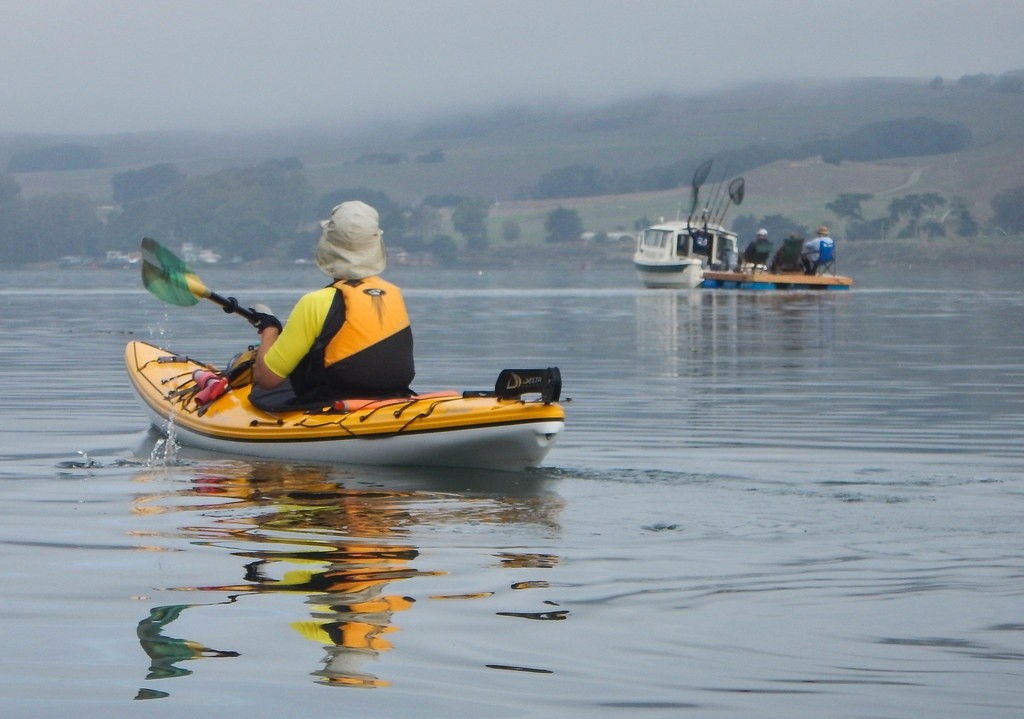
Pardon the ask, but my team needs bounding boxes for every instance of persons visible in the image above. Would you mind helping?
[800,226,834,275]
[770,233,800,272]
[244,199,415,412]
[738,228,770,272]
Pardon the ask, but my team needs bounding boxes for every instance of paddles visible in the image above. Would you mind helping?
[139,236,257,322]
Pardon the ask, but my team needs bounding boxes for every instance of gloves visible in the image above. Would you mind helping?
[246,303,283,335]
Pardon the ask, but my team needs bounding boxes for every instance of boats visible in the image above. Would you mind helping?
[632,220,740,288]
[696,256,855,295]
[121,337,568,471]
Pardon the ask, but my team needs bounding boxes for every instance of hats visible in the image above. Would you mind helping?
[315,201,386,280]
[757,229,768,236]
[816,227,828,234]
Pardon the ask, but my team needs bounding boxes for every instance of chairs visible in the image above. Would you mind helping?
[733,251,771,272]
[769,236,805,275]
[801,241,836,277]
